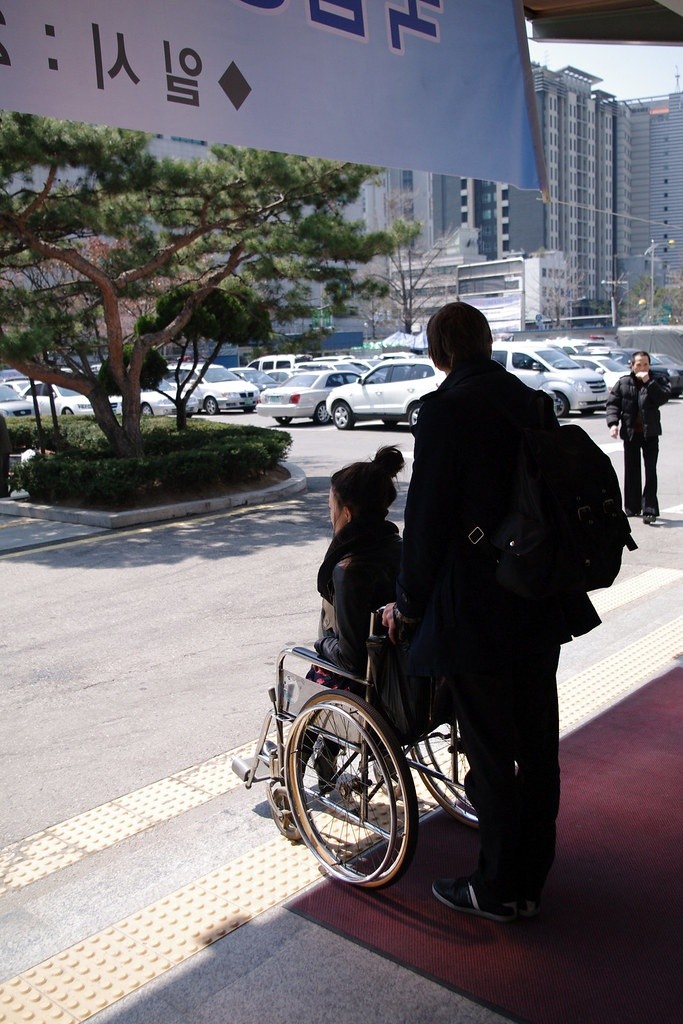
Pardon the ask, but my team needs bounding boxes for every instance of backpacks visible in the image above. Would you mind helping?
[498,392,636,598]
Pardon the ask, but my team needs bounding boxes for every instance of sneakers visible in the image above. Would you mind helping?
[431,875,515,921]
[516,899,540,916]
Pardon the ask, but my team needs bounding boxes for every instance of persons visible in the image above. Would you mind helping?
[606,352,671,524]
[258,447,404,791]
[379,302,562,920]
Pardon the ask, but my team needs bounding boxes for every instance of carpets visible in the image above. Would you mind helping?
[282,667,683,1024]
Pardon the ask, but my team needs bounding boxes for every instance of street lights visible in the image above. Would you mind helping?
[639,239,675,308]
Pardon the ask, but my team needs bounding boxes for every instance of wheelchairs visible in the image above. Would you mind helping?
[231,604,523,891]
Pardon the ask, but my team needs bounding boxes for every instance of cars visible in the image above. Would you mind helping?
[256,369,361,425]
[0,337,682,420]
[325,356,448,429]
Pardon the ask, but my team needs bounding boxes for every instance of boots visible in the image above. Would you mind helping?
[261,740,315,785]
[311,736,339,795]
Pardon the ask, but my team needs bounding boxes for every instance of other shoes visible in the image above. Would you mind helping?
[642,513,656,524]
